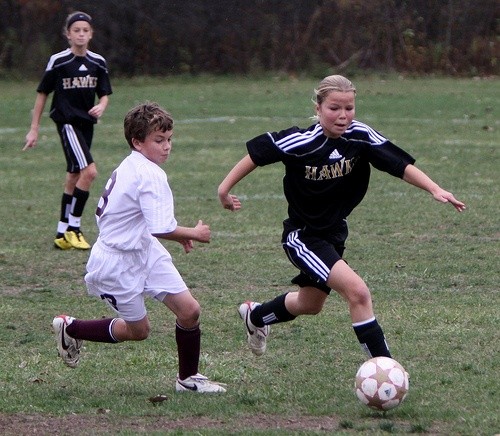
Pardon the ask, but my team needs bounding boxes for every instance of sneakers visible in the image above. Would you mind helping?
[54,234,76,250]
[63,229,93,249]
[175,370,227,393]
[52,314,81,368]
[239,300,270,356]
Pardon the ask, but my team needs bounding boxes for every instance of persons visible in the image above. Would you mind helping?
[52,102,229,394]
[218,74,465,383]
[21,10,113,252]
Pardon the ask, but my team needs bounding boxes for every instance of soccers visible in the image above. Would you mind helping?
[354,355,409,412]
